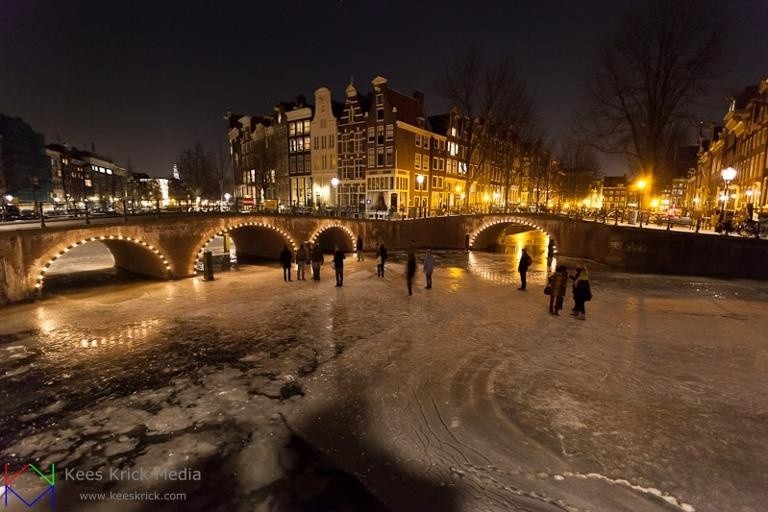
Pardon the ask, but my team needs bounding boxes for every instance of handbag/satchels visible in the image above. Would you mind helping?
[544,287,552,295]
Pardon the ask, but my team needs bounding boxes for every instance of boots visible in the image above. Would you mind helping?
[571,311,585,320]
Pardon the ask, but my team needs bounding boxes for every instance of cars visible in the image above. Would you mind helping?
[0,203,49,222]
[607,209,692,225]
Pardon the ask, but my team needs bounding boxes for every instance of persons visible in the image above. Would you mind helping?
[548,238,554,257]
[406,254,415,296]
[408,240,416,256]
[548,261,593,320]
[280,235,387,286]
[517,249,528,291]
[423,250,434,288]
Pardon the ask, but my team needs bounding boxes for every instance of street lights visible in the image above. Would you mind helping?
[331,177,339,217]
[416,175,425,218]
[714,164,737,233]
